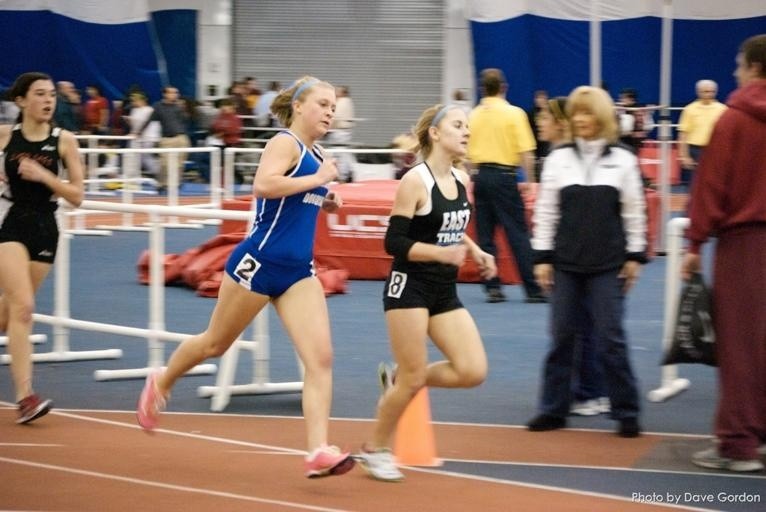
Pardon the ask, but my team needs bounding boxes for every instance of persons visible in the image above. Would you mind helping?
[1,72,84,425]
[680,34,766,474]
[2,78,353,194]
[467,69,546,304]
[679,79,730,184]
[527,86,649,438]
[538,95,608,417]
[139,74,359,480]
[356,104,498,484]
[619,89,654,143]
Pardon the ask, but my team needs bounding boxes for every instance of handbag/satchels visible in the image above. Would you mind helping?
[661,272,718,368]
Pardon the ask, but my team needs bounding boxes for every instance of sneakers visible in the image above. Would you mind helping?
[525,292,551,302]
[529,397,766,472]
[486,288,506,303]
[15,395,55,424]
[137,368,171,429]
[303,361,406,482]
